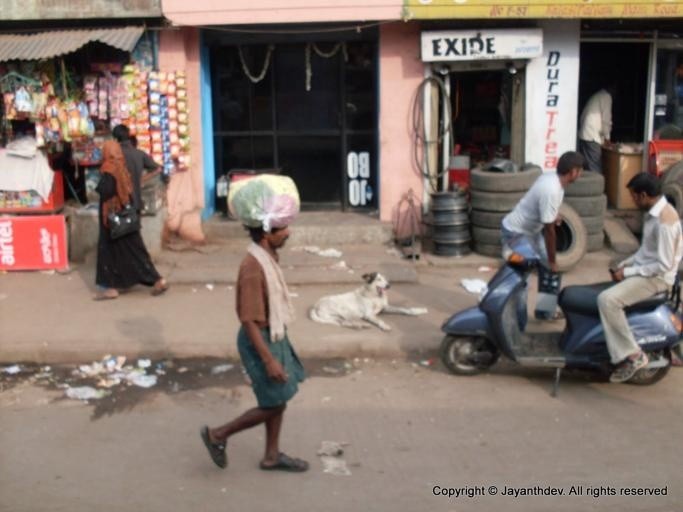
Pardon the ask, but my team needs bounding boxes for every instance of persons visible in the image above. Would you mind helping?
[93,125,168,300]
[579,81,621,173]
[48,131,79,201]
[597,172,683,383]
[502,151,585,319]
[199,224,308,472]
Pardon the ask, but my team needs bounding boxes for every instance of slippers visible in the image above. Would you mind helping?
[92,291,117,300]
[150,283,168,296]
[201,424,228,470]
[259,453,308,471]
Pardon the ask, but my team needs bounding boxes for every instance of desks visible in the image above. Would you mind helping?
[0,212,72,270]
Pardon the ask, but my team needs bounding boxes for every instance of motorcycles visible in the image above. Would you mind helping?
[435,233,681,386]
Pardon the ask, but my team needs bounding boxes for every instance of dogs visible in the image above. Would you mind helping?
[310,272,428,332]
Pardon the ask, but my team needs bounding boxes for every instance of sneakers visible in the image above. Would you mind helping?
[609,352,648,383]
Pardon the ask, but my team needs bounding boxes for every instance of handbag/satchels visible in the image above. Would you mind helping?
[107,204,140,239]
